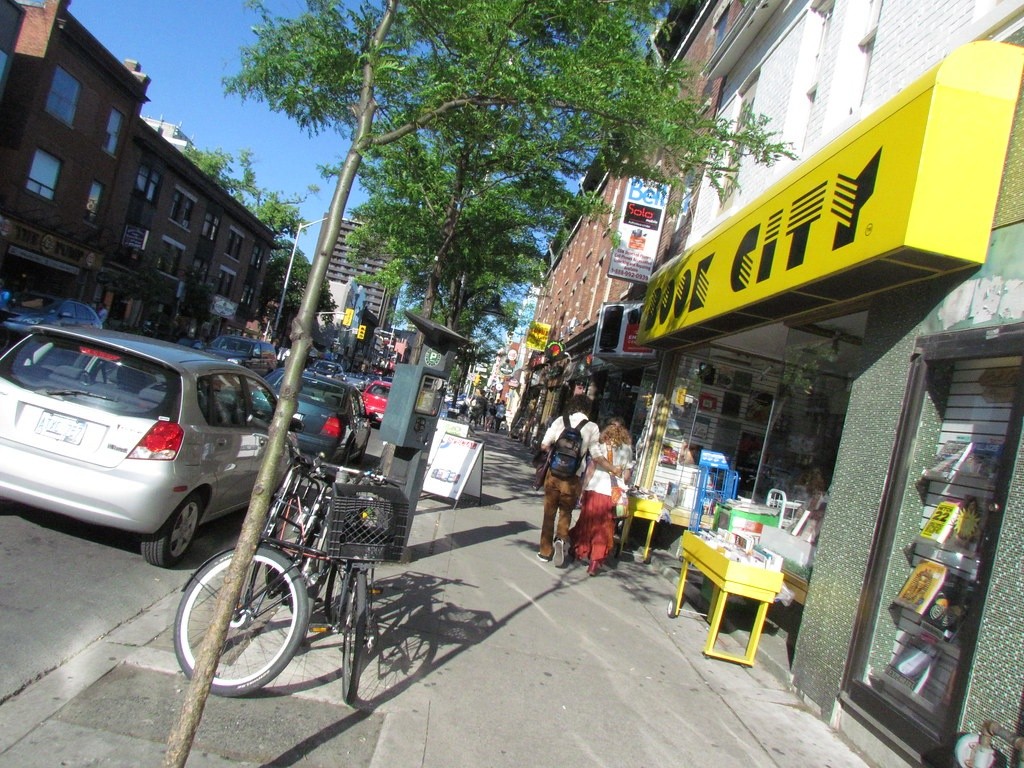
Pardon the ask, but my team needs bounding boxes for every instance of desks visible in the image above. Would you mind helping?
[614,494,663,563]
[668,530,785,668]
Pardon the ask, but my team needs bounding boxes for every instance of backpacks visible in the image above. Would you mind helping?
[549,413,590,480]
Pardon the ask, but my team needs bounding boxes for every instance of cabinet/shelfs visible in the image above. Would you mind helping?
[871,467,998,714]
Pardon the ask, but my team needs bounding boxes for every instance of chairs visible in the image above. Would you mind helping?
[298,385,341,406]
[49,365,166,410]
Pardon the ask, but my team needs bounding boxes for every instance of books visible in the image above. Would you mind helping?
[657,386,717,469]
[895,440,1005,642]
[694,528,784,573]
[791,508,824,542]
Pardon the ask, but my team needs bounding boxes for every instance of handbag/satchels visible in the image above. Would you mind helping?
[489,405,496,415]
[501,416,506,421]
[616,491,628,517]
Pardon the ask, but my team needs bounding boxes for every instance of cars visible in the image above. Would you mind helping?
[348,367,468,416]
[359,380,393,426]
[204,334,278,378]
[0,325,305,568]
[0,291,102,345]
[306,360,346,384]
[263,368,371,469]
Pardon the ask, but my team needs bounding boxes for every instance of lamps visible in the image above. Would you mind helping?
[756,365,774,382]
[826,329,841,363]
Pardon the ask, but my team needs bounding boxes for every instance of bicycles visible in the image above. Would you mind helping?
[172,431,333,698]
[312,455,408,705]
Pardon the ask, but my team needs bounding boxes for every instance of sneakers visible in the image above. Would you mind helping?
[537,552,548,562]
[553,538,564,567]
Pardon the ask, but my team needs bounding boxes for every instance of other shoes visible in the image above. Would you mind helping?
[487,429,489,431]
[484,429,486,431]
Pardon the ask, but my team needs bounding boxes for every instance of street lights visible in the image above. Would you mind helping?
[446,291,507,416]
[273,217,348,336]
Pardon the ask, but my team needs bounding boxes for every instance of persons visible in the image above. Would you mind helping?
[476,390,506,433]
[536,394,623,567]
[97,302,108,323]
[569,417,636,575]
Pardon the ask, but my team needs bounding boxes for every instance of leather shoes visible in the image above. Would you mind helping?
[587,560,598,576]
